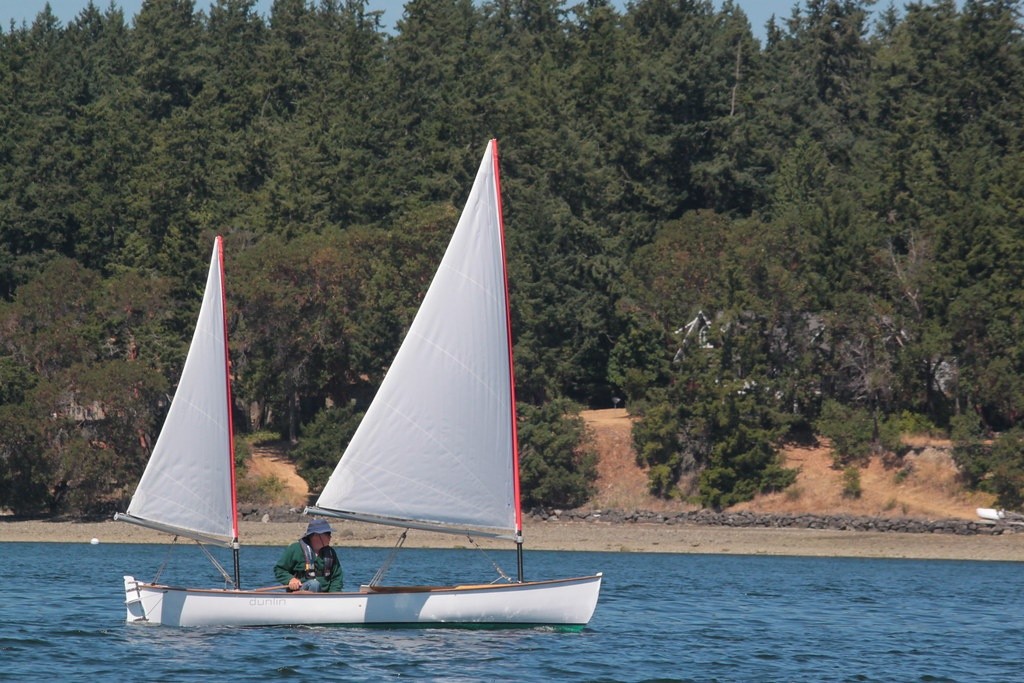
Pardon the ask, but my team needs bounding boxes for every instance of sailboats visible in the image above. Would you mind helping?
[110,139,601,638]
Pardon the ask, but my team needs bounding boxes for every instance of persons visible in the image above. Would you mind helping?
[274,517,344,594]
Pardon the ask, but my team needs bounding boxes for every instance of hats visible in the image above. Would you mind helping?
[297,519,337,540]
